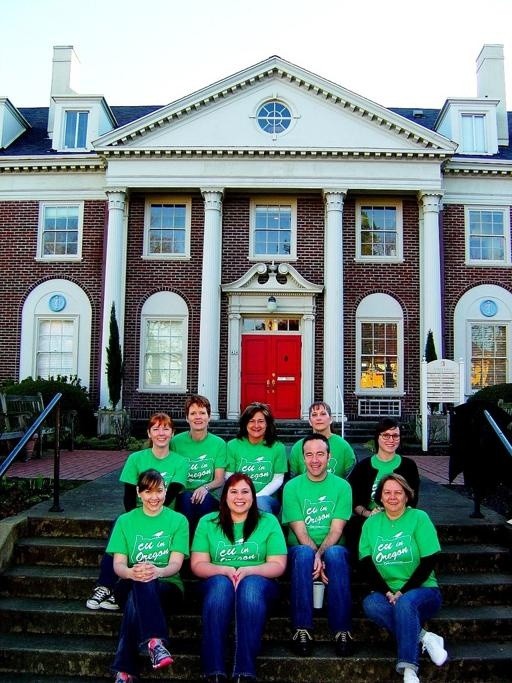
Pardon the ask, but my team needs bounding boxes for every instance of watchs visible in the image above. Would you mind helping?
[202,484,211,492]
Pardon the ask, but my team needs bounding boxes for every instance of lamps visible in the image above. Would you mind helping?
[255,259,289,285]
[266,295,278,314]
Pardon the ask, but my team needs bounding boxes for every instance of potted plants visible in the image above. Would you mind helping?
[414,328,450,445]
[94,300,131,439]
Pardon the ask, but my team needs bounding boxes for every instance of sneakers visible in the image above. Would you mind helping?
[115,672,132,682]
[293,629,312,658]
[87,586,110,610]
[148,637,174,668]
[403,668,421,682]
[421,632,449,666]
[335,630,354,657]
[100,595,121,611]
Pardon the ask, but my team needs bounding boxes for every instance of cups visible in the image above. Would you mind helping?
[138,562,150,582]
[313,581,325,609]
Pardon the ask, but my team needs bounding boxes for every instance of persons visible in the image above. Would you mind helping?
[345,419,421,550]
[357,472,449,683]
[289,401,357,478]
[279,433,356,656]
[216,400,288,516]
[86,410,189,609]
[170,392,227,542]
[103,470,190,683]
[187,473,288,682]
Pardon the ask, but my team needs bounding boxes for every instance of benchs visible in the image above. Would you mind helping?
[0,390,78,457]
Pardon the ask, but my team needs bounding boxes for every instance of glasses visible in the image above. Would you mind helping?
[378,432,398,441]
